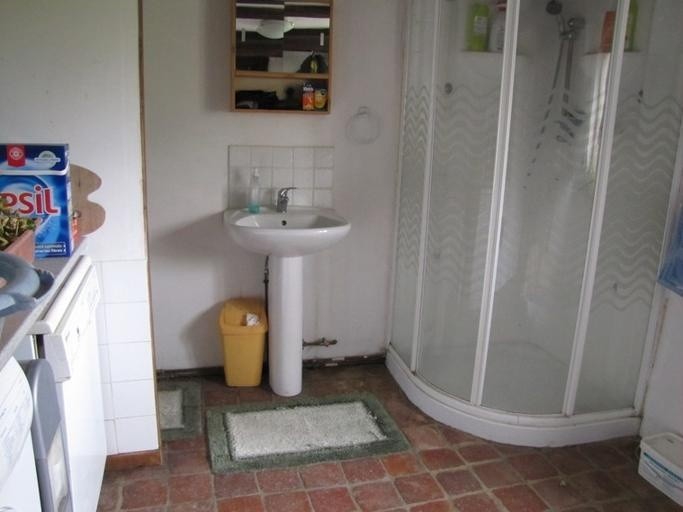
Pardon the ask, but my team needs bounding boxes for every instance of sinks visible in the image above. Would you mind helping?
[223,205,352,258]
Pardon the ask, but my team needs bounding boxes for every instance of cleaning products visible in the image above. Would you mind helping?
[488,0,507,53]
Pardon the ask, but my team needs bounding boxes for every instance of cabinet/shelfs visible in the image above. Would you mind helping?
[227,0,332,116]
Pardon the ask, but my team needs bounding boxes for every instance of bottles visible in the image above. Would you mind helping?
[248,168,260,214]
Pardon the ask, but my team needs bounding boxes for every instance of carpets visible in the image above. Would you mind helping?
[157,377,411,476]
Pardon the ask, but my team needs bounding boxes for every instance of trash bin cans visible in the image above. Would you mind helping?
[217,296,270,389]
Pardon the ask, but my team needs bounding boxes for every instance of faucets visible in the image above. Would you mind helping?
[277,187,298,213]
